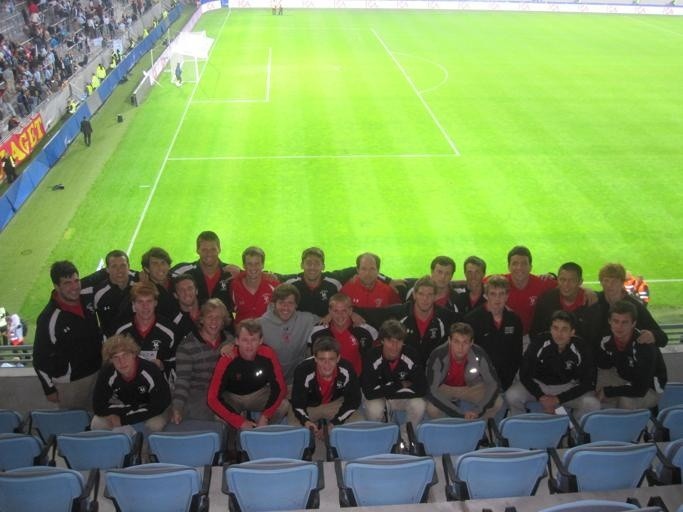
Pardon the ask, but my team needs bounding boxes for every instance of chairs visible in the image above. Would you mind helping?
[0,382,682,511]
[0,1,161,149]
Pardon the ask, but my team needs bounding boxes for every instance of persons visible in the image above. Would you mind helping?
[272,0,277,15]
[0,0,183,192]
[278,0,283,15]
